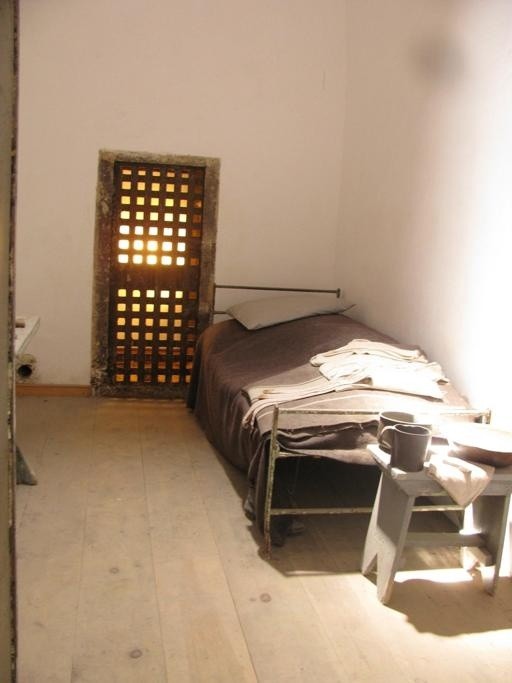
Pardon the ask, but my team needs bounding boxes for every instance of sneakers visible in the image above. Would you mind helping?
[244,486,305,534]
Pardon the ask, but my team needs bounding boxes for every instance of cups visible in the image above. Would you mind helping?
[378,423,432,472]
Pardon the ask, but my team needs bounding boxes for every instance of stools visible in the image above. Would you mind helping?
[361,442,512,603]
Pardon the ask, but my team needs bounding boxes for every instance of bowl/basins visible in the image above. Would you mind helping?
[378,411,432,451]
[446,422,512,467]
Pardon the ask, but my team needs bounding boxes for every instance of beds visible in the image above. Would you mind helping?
[185,281,493,560]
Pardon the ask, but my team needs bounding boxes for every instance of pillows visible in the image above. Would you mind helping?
[224,292,356,331]
